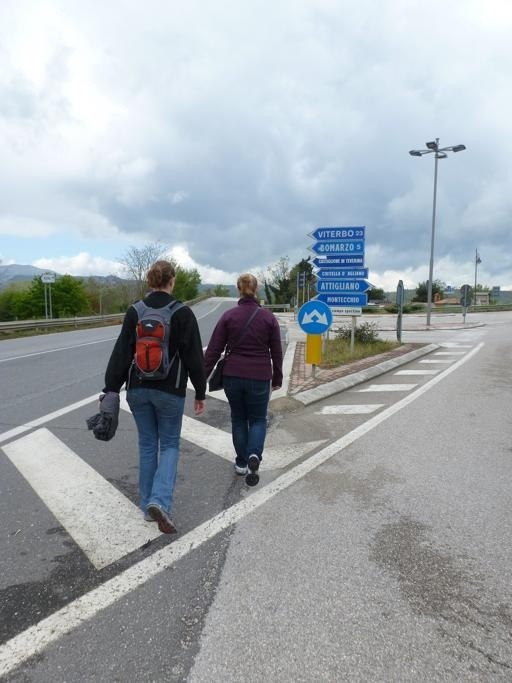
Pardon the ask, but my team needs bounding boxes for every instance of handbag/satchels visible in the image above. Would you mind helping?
[209,358,225,392]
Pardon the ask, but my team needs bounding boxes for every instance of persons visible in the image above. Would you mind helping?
[202,273,283,487]
[103,257,207,533]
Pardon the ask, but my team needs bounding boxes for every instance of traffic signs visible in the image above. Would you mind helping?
[329,307,362,316]
[311,280,372,294]
[312,266,368,280]
[310,293,368,307]
[306,242,365,254]
[308,255,362,268]
[307,226,365,241]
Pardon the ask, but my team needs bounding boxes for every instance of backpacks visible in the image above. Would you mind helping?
[130,298,189,380]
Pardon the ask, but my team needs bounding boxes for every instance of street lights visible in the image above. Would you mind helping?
[409,137,465,326]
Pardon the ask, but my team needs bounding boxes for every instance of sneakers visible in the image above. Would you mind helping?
[144,504,179,534]
[234,454,260,486]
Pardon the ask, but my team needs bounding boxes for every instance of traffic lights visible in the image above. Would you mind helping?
[299,273,304,287]
